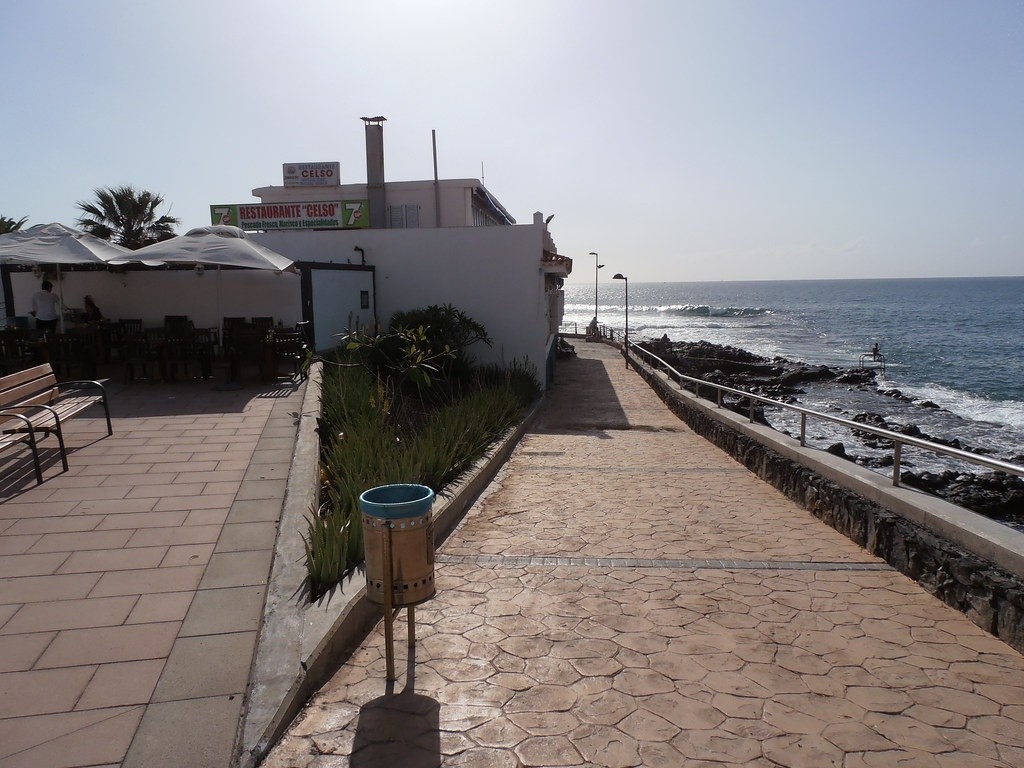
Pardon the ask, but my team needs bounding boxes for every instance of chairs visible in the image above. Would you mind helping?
[0,316,312,382]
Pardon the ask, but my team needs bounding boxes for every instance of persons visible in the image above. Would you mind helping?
[32,281,60,337]
[873,343,881,357]
[80,298,102,323]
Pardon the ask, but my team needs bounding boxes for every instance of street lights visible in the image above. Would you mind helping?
[590,252,605,319]
[612,273,629,369]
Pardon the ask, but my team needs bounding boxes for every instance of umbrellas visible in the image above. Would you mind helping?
[108,226,296,347]
[1,222,136,335]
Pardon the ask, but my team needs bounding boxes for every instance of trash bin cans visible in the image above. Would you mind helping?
[359,485,436,605]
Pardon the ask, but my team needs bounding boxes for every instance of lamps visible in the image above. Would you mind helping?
[32,264,42,279]
[195,262,205,275]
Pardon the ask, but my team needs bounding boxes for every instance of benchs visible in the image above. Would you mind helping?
[0,414,43,485]
[0,363,114,473]
